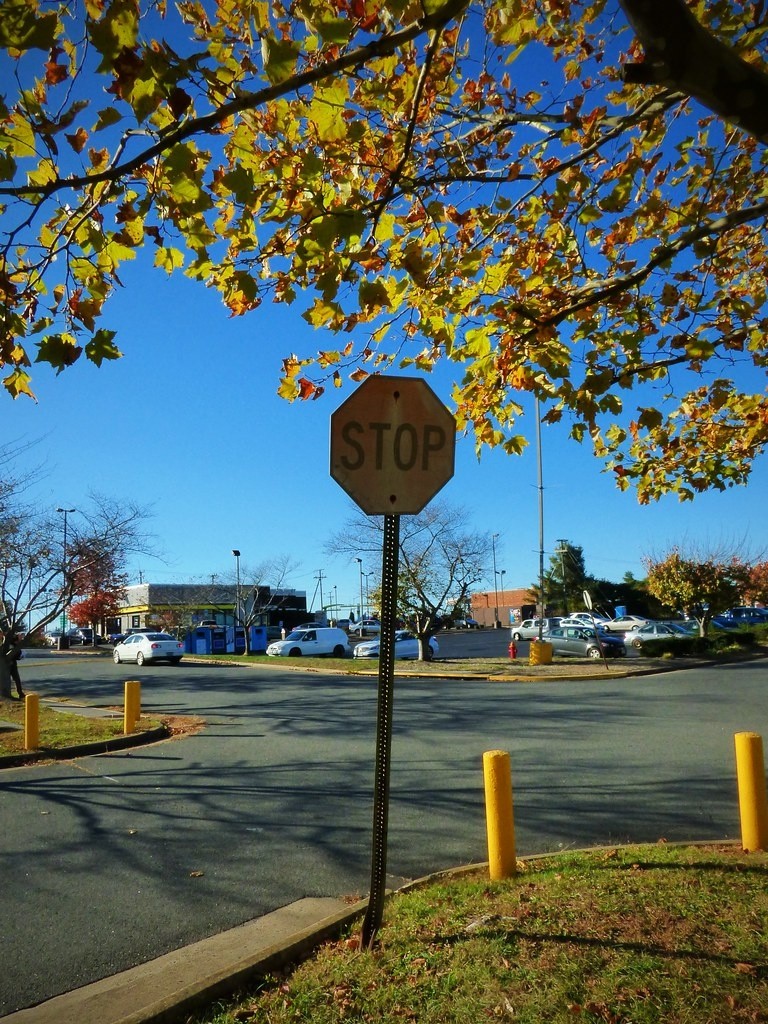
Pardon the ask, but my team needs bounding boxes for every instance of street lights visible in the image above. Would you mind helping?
[353,558,365,630]
[361,572,374,620]
[495,570,506,604]
[232,550,241,626]
[42,587,54,633]
[492,533,499,620]
[333,585,338,625]
[56,507,75,635]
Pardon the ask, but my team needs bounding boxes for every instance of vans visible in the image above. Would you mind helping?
[266,628,351,657]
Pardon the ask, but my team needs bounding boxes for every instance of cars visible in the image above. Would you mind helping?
[337,619,352,628]
[112,632,184,665]
[455,618,478,629]
[353,630,439,661]
[510,607,768,658]
[349,619,381,635]
[110,635,123,643]
[45,632,68,645]
[67,628,101,646]
[292,622,327,631]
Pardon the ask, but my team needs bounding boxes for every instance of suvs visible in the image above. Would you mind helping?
[200,619,217,627]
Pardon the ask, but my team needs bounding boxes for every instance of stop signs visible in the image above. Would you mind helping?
[329,373,456,514]
